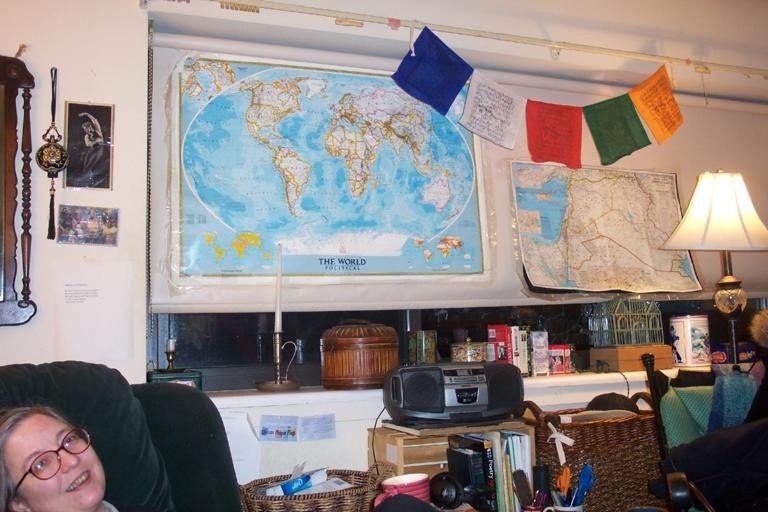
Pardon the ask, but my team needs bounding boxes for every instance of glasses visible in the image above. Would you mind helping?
[13,427,91,498]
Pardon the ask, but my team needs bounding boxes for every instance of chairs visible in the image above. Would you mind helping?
[0,359,244,511]
[640,353,767,512]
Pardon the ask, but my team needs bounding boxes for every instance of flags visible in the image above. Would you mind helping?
[459,69,530,152]
[629,63,686,147]
[392,26,472,116]
[524,97,586,171]
[579,91,653,167]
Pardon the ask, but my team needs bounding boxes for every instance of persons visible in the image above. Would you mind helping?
[56,207,118,244]
[77,110,104,178]
[0,402,120,511]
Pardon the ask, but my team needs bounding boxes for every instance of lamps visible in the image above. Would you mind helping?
[658,168,768,358]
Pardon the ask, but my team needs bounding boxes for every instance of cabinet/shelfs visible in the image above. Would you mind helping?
[367,420,537,477]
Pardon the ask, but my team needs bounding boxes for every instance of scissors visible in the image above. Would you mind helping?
[577,465,594,499]
[555,466,571,501]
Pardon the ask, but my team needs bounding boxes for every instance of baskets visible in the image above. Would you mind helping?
[521,391,670,511]
[236,459,395,512]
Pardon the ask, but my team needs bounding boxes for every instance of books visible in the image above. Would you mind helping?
[444,430,538,512]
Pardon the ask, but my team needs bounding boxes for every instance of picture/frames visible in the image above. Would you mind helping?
[62,101,115,191]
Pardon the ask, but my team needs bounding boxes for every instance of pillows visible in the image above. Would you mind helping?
[707,363,758,433]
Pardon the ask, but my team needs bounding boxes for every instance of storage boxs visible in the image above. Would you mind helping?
[590,343,675,373]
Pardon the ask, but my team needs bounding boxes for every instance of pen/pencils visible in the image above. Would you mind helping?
[551,486,594,508]
[533,489,547,508]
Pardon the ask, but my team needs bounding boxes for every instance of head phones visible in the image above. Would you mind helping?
[431,472,492,510]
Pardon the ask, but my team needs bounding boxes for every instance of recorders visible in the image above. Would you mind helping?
[384,361,524,431]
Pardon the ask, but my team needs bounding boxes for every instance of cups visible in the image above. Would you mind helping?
[542,502,586,512]
[373,472,432,509]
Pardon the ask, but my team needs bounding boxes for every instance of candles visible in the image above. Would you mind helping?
[274,242,283,333]
